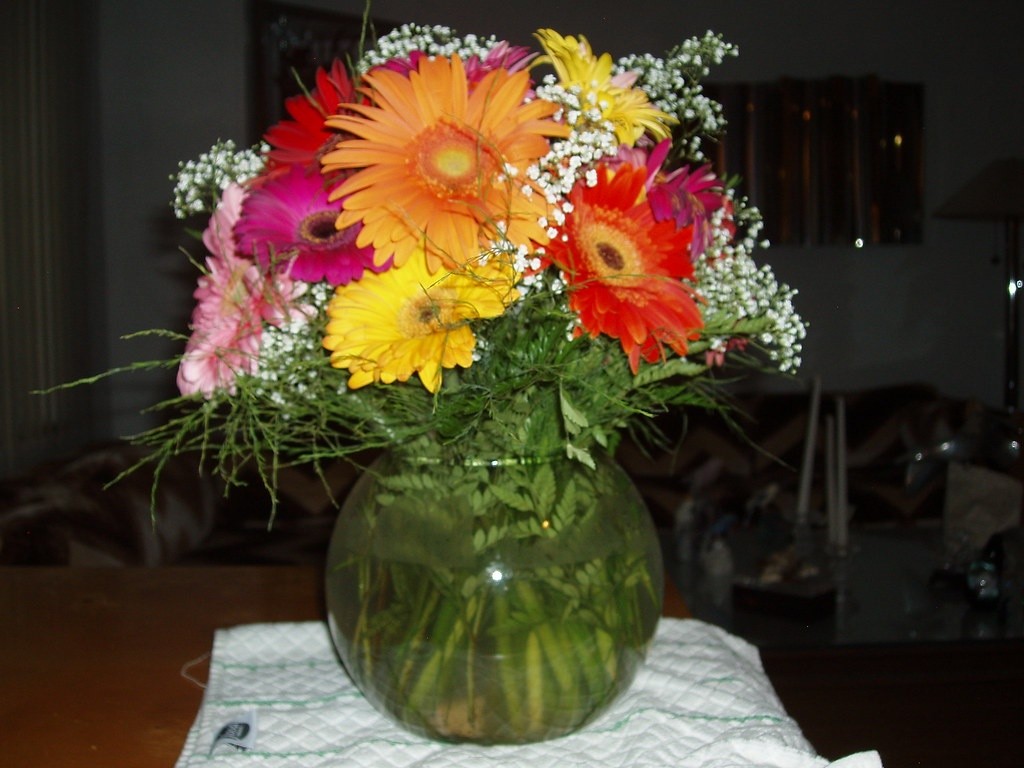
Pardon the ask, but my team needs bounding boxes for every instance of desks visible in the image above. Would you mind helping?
[0,562,674,768]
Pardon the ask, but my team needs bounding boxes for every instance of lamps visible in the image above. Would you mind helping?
[935,156,1023,416]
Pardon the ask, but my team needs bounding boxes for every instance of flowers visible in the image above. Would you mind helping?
[36,2,813,537]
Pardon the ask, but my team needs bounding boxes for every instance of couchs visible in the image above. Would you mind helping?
[6,391,958,570]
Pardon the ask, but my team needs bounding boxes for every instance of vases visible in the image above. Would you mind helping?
[327,435,668,746]
